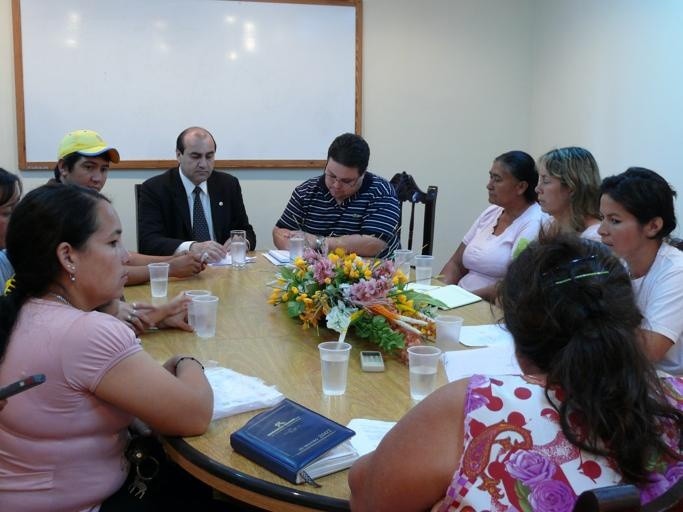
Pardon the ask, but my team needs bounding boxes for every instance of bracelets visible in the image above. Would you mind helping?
[173,356,205,374]
[316,233,325,253]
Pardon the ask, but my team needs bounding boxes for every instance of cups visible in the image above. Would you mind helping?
[230,229,246,268]
[393,250,413,279]
[318,341,351,395]
[185,290,212,325]
[191,295,219,337]
[290,231,304,261]
[414,255,433,287]
[148,262,170,298]
[406,345,442,400]
[435,315,463,350]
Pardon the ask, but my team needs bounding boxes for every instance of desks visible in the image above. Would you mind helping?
[116,248,507,512]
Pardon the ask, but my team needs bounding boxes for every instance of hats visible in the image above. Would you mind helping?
[57,130,119,164]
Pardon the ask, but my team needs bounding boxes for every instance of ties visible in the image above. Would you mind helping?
[191,186,211,242]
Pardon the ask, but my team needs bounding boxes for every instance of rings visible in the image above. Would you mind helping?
[132,309,136,315]
[128,315,133,321]
[133,302,137,307]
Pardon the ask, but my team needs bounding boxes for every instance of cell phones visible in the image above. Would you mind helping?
[0,373,46,401]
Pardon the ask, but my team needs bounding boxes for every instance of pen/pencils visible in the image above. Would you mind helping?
[201,252,209,264]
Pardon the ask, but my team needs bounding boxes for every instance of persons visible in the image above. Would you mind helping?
[0,181,215,512]
[436,150,550,311]
[597,166,682,373]
[272,131,402,262]
[0,167,211,337]
[139,126,257,265]
[535,146,599,243]
[347,216,683,511]
[50,125,120,190]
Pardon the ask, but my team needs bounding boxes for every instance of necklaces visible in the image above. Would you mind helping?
[49,290,72,305]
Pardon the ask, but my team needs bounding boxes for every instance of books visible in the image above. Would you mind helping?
[229,397,359,486]
[423,284,482,310]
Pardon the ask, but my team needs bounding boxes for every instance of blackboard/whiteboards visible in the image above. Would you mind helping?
[12,0,362,170]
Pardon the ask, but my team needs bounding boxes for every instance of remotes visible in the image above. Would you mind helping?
[359,350,385,372]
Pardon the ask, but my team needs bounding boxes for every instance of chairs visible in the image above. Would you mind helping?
[570,474,683,512]
[134,182,142,254]
[667,236,683,251]
[390,170,438,257]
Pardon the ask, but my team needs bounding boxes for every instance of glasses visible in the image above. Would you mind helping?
[322,162,361,190]
